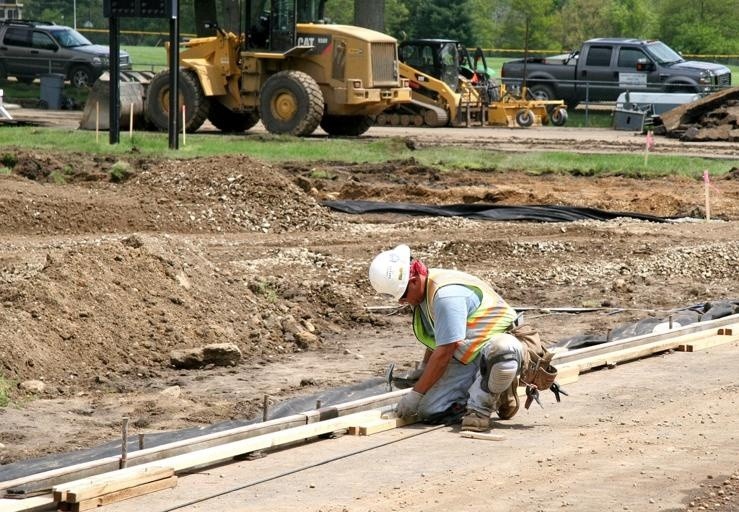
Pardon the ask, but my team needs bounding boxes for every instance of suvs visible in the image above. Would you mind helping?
[0,18,134,89]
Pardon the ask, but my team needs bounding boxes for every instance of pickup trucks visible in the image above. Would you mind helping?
[501,36,734,111]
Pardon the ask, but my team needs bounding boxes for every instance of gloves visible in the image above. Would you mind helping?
[393,389,426,422]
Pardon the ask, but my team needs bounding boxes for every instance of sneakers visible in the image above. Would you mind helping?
[459,407,494,433]
[494,375,523,422]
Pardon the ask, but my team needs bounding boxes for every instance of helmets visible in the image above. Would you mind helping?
[366,243,413,307]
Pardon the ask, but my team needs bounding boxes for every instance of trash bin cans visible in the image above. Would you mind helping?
[36,73,67,110]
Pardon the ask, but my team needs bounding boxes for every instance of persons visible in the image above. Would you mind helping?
[367,242,543,432]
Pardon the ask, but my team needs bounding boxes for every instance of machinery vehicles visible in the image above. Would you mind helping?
[74,0,415,138]
[373,37,504,128]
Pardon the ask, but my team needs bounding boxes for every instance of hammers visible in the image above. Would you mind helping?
[385,363,419,392]
